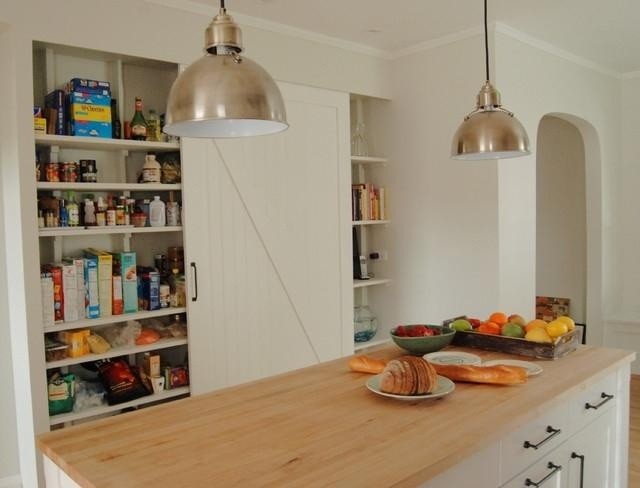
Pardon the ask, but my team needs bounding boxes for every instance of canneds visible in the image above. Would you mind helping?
[61,161,77,182]
[45,161,60,182]
[167,201,181,226]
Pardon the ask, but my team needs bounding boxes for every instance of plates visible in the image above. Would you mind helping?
[424,352,482,365]
[366,372,454,401]
[484,359,543,377]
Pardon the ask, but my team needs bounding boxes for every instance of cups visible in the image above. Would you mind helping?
[151,376,164,394]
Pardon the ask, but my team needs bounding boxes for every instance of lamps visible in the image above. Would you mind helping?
[449,0,533,161]
[162,0,289,139]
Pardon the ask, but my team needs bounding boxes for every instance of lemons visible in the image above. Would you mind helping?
[524,312,575,344]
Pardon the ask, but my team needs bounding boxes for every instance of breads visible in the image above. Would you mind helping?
[380,355,436,395]
[347,355,530,386]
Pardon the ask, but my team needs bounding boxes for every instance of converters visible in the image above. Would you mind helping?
[370,253,379,259]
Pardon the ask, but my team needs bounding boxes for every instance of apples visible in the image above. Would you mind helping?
[499,314,527,338]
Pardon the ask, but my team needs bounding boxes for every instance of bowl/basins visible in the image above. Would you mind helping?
[390,325,457,356]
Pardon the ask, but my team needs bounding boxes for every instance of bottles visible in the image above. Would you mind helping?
[142,152,161,183]
[131,96,148,142]
[39,194,178,229]
[146,109,161,141]
[124,120,131,139]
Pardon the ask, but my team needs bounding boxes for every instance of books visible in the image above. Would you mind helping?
[350,182,386,222]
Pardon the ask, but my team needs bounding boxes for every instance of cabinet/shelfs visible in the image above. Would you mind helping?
[348,91,393,349]
[413,364,630,488]
[31,37,191,424]
